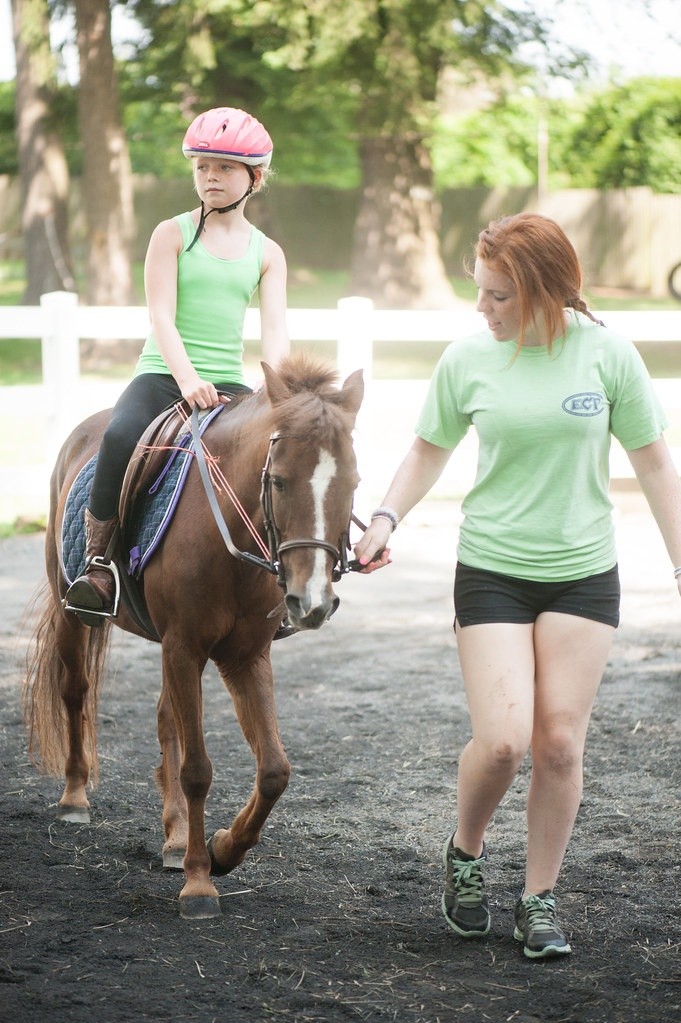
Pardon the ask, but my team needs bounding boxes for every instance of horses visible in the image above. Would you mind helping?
[14,354,364,919]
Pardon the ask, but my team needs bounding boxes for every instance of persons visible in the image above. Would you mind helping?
[354,213,681,959]
[63,106,292,629]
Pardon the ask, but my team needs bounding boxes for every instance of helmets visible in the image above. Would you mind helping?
[182,106,274,171]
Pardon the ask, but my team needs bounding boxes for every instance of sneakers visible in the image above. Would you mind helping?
[441,829,492,936]
[514,887,572,959]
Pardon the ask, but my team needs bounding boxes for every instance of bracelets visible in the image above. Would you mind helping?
[674,567,681,578]
[370,507,399,533]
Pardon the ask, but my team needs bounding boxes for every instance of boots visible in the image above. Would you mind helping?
[66,507,121,629]
